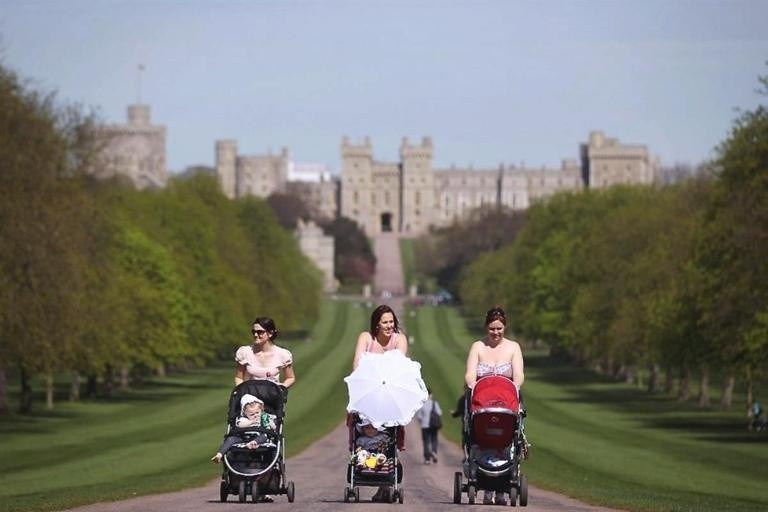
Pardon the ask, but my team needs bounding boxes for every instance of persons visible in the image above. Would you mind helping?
[448,382,471,462]
[234,315,295,501]
[210,393,277,465]
[417,388,444,463]
[465,307,525,504]
[355,416,389,468]
[352,304,409,502]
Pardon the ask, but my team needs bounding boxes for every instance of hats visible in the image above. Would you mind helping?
[355,417,386,433]
[239,394,265,417]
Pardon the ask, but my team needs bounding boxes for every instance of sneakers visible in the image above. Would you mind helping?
[495,491,508,506]
[482,491,494,504]
[424,451,438,465]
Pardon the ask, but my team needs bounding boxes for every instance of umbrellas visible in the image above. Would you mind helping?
[344,349,429,429]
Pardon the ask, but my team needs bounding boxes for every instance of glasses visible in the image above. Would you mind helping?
[252,328,267,336]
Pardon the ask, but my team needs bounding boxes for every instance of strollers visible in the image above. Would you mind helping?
[341,412,410,506]
[214,377,299,501]
[450,371,537,508]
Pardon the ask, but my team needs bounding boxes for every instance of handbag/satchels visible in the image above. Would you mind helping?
[429,402,442,429]
